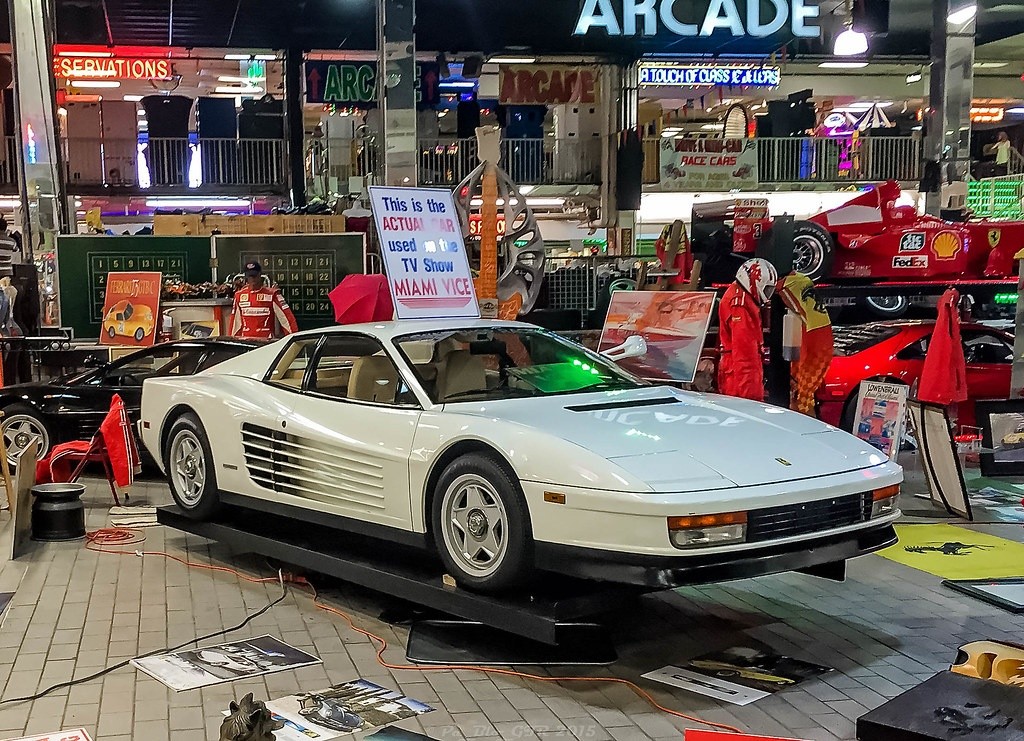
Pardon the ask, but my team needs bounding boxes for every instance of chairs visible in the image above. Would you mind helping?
[438,350,487,404]
[346,355,396,404]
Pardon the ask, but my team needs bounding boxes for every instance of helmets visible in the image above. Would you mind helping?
[735,258,778,306]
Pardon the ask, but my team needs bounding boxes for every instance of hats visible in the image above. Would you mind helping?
[244,261,261,276]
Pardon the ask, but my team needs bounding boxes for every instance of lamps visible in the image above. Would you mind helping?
[834,22,868,55]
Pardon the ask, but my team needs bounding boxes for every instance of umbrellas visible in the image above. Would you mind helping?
[329,274,395,325]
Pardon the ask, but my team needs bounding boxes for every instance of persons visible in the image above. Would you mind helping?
[992,131,1011,175]
[227,260,300,340]
[716,257,777,403]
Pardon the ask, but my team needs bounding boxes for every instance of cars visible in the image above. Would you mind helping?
[136,320,905,598]
[0,336,266,479]
[814,320,1024,435]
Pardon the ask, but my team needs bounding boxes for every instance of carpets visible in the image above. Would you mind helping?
[872,523,1024,580]
